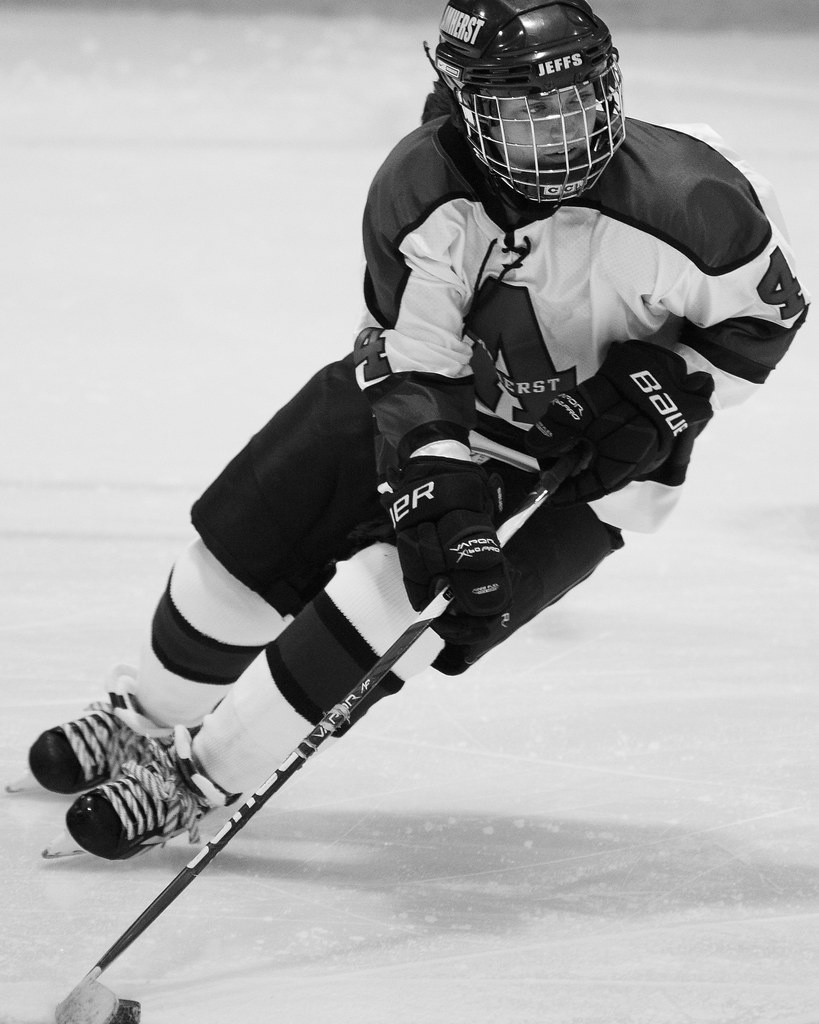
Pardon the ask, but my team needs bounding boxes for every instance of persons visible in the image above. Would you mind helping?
[5,1,812,864]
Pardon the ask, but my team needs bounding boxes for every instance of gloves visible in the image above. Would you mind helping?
[380,456,513,614]
[529,339,714,506]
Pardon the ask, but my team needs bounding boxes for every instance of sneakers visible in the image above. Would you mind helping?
[40,721,241,859]
[5,683,225,796]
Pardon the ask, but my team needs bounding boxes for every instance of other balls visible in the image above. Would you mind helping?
[110,998,142,1024]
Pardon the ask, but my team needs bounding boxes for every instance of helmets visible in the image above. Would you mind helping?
[434,0,626,211]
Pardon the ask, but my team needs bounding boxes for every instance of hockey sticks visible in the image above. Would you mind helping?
[52,448,583,1023]
